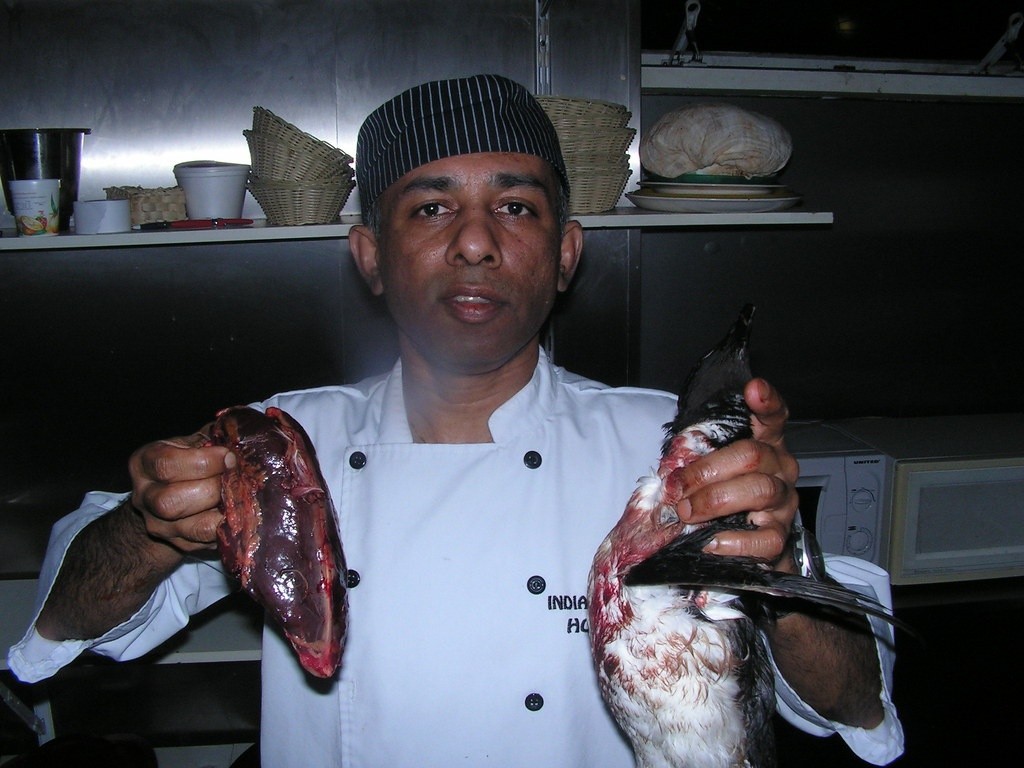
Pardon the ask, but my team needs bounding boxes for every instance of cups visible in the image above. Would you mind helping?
[1,127,93,233]
[7,178,62,238]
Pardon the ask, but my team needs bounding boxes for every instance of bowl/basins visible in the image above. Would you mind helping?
[172,159,252,220]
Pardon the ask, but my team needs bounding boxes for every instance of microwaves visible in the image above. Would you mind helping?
[779,421,888,567]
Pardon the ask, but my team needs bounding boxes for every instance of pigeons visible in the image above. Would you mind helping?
[586,301,915,768]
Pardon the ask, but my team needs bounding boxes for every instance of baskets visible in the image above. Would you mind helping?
[243,106,356,226]
[533,95,638,213]
[102,187,186,230]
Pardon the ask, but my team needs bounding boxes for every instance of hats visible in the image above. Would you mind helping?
[355,74,570,225]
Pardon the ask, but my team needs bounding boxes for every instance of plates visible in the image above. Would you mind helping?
[625,174,803,211]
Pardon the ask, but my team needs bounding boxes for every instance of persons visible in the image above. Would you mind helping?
[7,74,903,768]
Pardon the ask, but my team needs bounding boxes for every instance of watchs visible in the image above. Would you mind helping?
[788,523,826,581]
